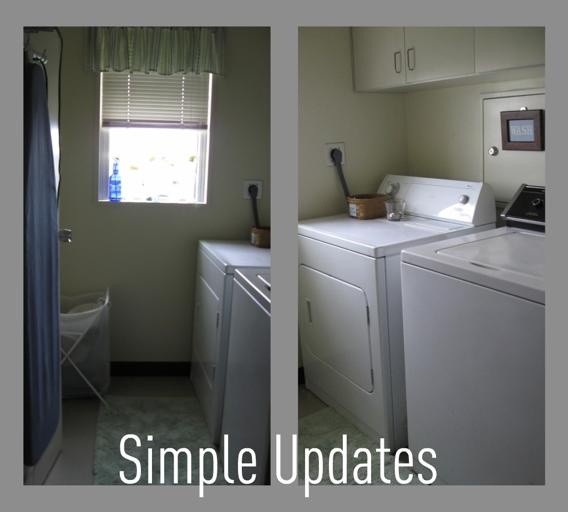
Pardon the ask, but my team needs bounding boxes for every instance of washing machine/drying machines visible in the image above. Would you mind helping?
[191,236,271,447]
[297,170,498,454]
[400,182,544,484]
[213,265,271,481]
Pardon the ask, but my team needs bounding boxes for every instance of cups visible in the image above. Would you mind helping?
[384,200,405,221]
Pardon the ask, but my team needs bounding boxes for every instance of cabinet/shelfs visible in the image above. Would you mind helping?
[350,27,475,95]
[475,26,544,83]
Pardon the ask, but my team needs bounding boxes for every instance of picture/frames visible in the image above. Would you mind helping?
[499,110,544,152]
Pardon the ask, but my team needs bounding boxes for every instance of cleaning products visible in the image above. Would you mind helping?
[108,156,122,201]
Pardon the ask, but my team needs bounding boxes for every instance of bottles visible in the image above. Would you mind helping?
[108,163,121,202]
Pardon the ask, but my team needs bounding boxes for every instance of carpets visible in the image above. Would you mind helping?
[298,408,428,485]
[94,392,227,484]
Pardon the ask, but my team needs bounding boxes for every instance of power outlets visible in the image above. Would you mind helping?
[323,142,344,166]
[243,180,262,199]
